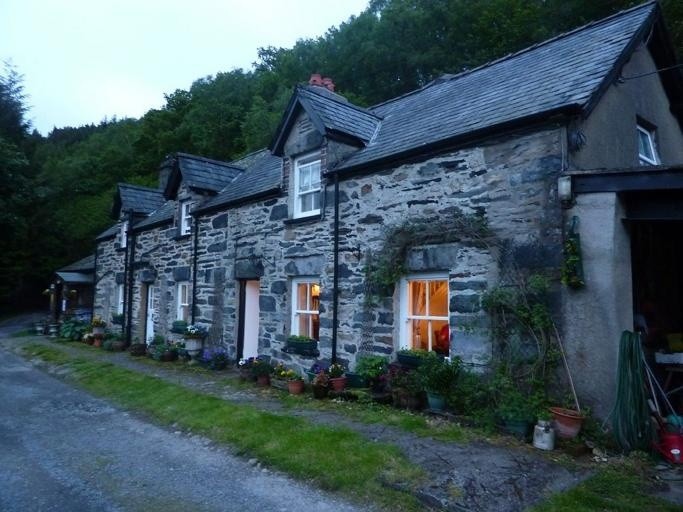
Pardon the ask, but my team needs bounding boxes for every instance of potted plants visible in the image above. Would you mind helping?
[32,313,226,370]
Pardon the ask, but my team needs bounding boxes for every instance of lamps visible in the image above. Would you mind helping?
[558,177,576,210]
[311,284,320,298]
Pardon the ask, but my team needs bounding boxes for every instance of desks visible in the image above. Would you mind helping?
[661,367,683,397]
